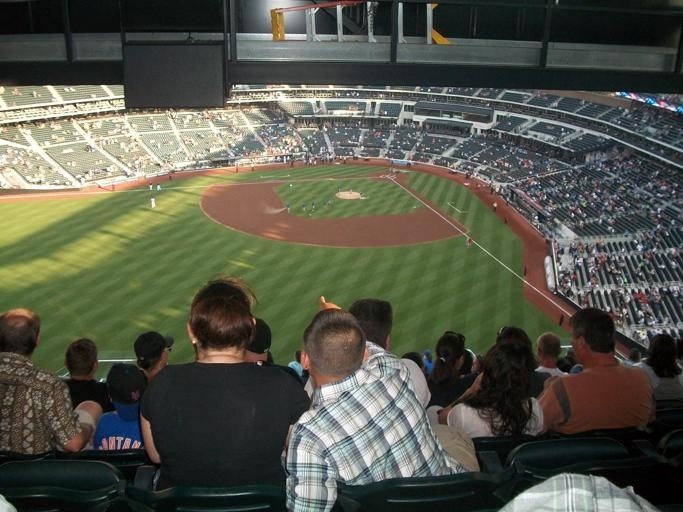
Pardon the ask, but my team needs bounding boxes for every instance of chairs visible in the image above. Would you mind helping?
[463,88,547,187]
[1,88,125,188]
[547,90,683,362]
[225,90,467,179]
[125,109,227,180]
[1,425,683,511]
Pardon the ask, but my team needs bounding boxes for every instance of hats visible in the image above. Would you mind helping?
[134,331,173,354]
